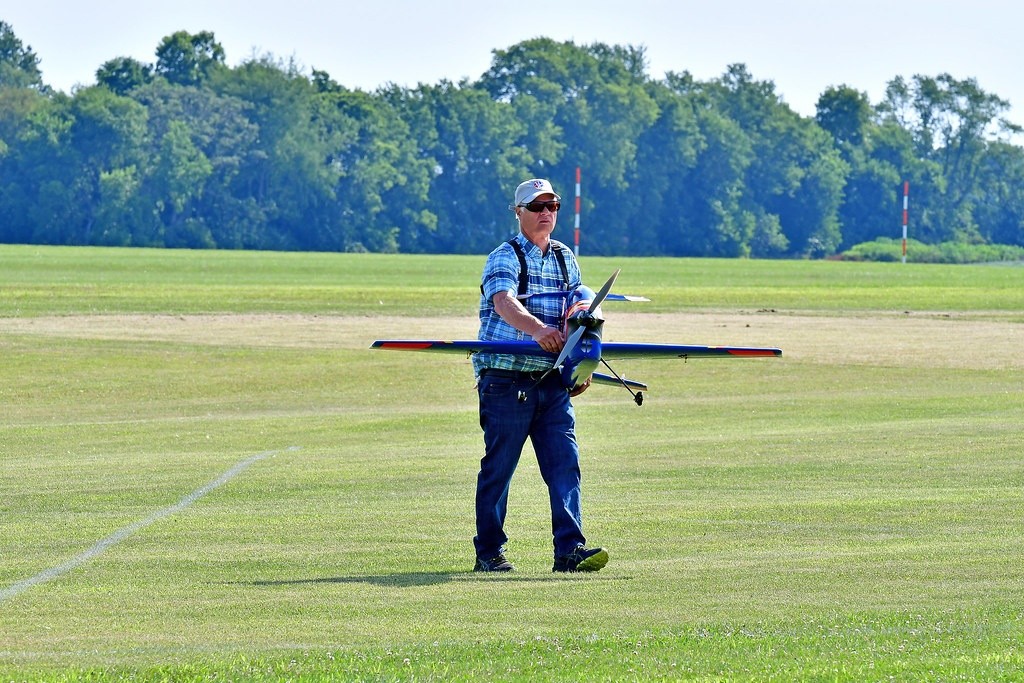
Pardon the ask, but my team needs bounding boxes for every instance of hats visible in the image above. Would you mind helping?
[508,179,561,211]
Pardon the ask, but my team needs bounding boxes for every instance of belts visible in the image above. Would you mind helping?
[484,369,559,381]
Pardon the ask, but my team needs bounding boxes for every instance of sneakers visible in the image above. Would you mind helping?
[553,546,608,572]
[474,554,513,572]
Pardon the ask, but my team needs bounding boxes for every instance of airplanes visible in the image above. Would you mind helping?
[368,268,783,406]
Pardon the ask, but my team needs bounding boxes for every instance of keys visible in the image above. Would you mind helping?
[518,390,525,403]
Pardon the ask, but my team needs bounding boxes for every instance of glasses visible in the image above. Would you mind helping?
[518,200,560,212]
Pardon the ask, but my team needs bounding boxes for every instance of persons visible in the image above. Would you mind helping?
[472,179,609,572]
